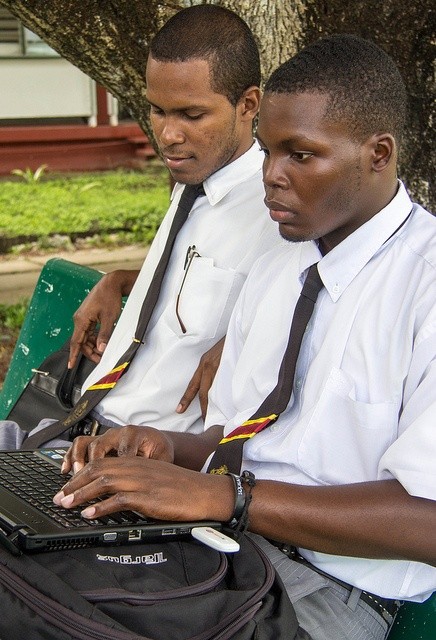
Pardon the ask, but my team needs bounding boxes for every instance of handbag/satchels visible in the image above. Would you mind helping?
[6,337,100,437]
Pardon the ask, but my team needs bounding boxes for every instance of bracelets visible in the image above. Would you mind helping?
[223,473,248,531]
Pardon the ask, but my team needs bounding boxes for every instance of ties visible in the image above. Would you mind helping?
[205,261,322,476]
[20,183,203,451]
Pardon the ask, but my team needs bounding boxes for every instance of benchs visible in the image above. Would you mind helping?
[0,257,434,639]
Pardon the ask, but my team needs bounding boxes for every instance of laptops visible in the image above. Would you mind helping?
[1,447,223,557]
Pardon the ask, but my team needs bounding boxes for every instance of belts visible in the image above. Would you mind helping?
[269,541,398,624]
[70,415,112,439]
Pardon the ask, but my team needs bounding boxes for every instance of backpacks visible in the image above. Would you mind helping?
[0,528,311,639]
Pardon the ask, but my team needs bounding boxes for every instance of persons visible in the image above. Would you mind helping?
[51,38,434,640]
[3,5,300,453]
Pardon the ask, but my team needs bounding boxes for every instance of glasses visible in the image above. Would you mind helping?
[175,245,200,333]
